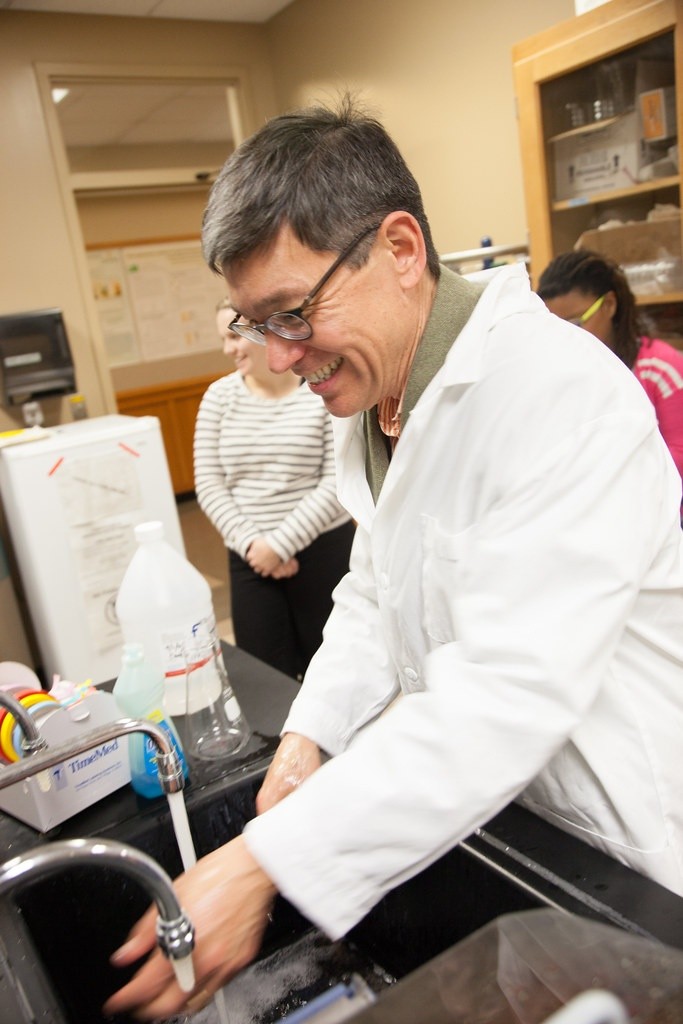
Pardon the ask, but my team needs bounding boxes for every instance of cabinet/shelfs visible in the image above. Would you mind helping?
[508,0,683,353]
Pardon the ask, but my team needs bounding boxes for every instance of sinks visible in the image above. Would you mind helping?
[0,743,681,1024]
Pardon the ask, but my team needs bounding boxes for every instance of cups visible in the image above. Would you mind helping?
[184,635,252,760]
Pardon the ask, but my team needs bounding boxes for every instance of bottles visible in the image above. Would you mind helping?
[115,521,214,716]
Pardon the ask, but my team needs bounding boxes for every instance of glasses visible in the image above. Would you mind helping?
[568,295,606,327]
[227,222,380,346]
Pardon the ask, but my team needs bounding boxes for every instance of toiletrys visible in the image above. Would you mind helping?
[109,640,188,796]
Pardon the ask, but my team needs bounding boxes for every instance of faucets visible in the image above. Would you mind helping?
[0,690,49,757]
[0,721,187,798]
[0,834,197,964]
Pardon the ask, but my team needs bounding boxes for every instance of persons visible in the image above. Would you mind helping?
[535,248,683,530]
[102,95,683,1022]
[192,296,358,683]
